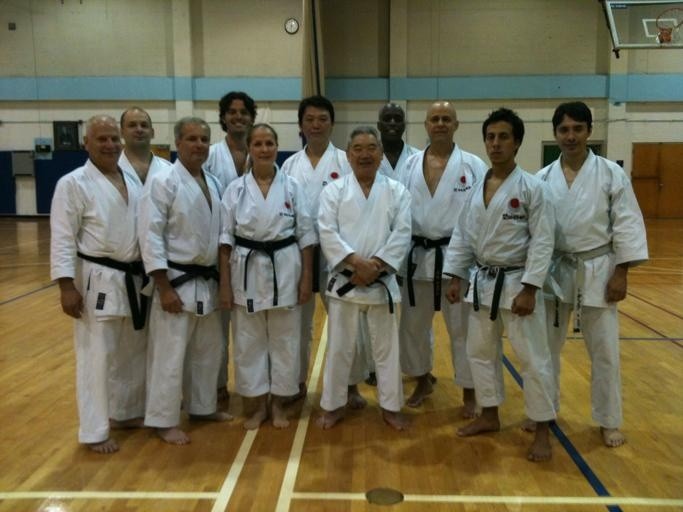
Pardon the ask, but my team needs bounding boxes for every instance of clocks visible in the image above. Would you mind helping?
[283,17,300,35]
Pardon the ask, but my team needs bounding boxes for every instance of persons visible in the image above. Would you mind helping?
[398,100,490,419]
[315,126,410,431]
[49,116,153,453]
[440,110,556,465]
[365,99,439,389]
[276,94,370,411]
[136,116,233,448]
[218,121,318,430]
[121,109,173,185]
[519,99,655,449]
[206,89,261,402]
